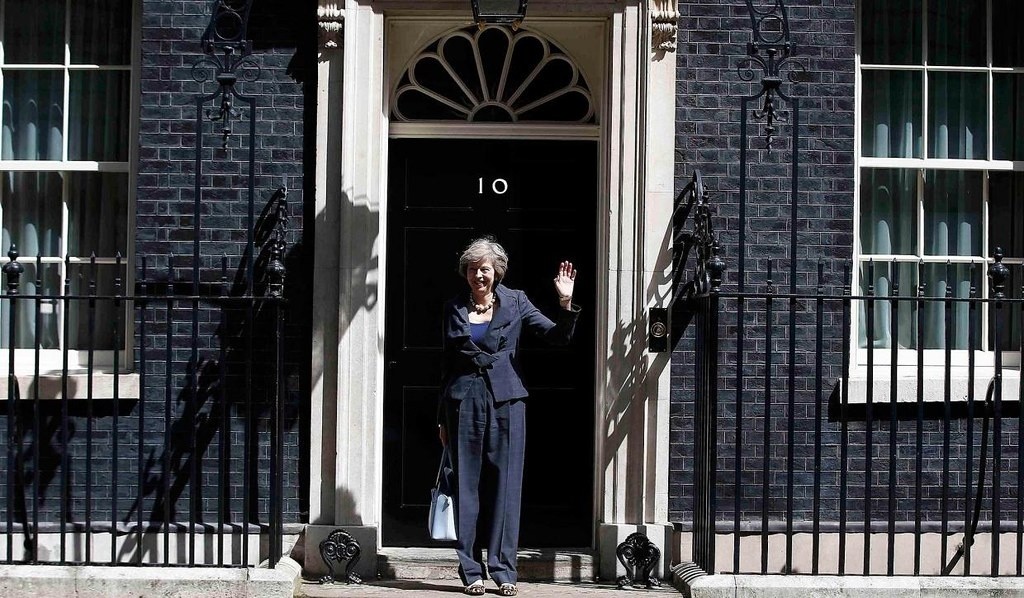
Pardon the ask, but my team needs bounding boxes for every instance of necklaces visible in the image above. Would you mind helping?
[470,292,496,312]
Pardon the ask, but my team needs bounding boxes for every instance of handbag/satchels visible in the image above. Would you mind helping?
[428,445,458,542]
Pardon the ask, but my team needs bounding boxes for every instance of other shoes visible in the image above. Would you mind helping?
[464,580,485,595]
[499,583,518,596]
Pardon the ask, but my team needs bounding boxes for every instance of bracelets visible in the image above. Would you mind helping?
[559,296,572,301]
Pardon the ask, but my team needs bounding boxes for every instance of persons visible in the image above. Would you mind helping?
[433,237,582,596]
